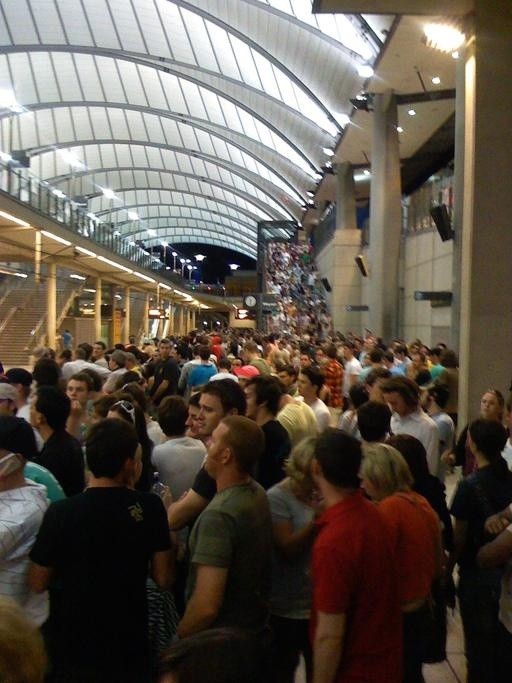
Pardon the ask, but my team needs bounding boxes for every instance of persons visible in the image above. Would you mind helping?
[266,239,332,335]
[0,324,512,547]
[175,415,276,650]
[0,600,49,682]
[27,418,176,682]
[476,503,512,683]
[0,414,51,639]
[266,436,327,683]
[159,628,272,683]
[384,434,457,683]
[308,428,407,682]
[358,443,444,683]
[448,418,512,682]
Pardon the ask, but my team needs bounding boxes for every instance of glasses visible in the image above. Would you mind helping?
[115,399,136,425]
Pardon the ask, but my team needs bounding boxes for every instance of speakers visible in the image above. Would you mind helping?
[320,278,332,292]
[428,205,454,242]
[354,255,369,279]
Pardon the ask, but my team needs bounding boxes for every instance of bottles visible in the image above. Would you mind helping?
[151,471,165,497]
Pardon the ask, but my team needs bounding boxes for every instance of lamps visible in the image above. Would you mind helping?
[283,88,384,210]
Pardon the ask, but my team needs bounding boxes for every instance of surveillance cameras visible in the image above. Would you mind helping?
[73,250,80,256]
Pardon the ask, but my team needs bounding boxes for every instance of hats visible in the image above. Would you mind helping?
[0,415,37,461]
[0,384,21,401]
[233,364,260,380]
[213,336,220,343]
[107,350,128,366]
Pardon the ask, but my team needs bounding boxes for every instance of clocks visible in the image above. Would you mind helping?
[244,295,258,308]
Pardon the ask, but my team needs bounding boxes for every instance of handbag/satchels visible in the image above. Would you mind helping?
[424,605,447,663]
